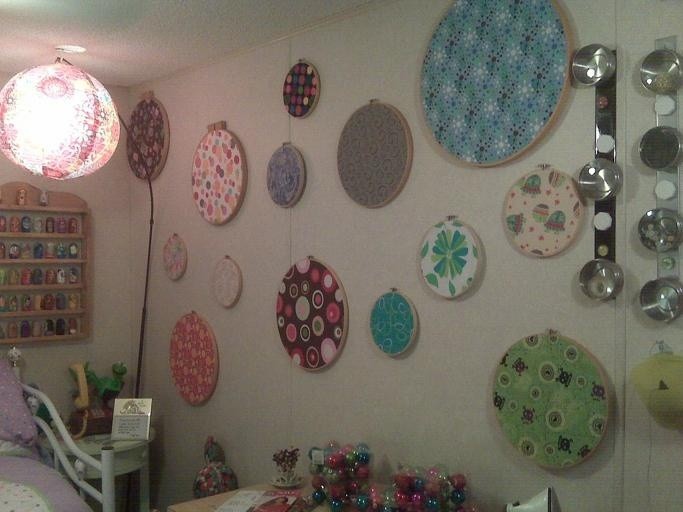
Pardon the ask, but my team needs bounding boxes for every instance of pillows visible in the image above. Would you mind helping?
[0,357,39,446]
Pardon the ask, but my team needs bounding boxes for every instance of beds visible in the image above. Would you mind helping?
[0,346,117,512]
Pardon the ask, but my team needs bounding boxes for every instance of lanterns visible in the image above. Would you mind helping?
[0,61,122,182]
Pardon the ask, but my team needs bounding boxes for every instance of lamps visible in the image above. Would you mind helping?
[0,59,154,398]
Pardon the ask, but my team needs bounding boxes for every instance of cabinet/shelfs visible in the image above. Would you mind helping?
[0,182,94,344]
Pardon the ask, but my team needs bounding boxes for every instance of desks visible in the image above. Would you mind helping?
[168,483,310,511]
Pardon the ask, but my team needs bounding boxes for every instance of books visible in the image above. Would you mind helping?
[214,490,302,512]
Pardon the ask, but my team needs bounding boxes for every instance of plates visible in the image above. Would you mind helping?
[269,477,303,489]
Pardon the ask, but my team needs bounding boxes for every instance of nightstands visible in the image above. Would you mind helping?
[38,423,156,512]
